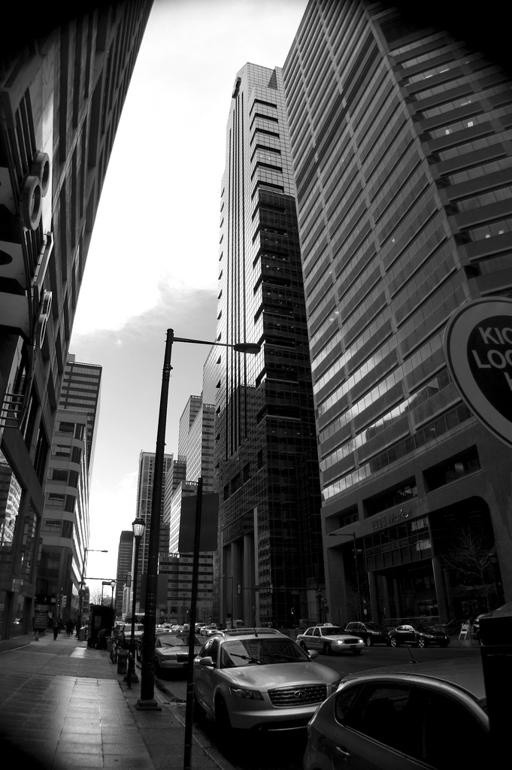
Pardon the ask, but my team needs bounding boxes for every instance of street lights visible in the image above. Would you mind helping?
[137,328,262,712]
[79,547,117,659]
[121,517,147,683]
[328,531,367,623]
[214,576,234,629]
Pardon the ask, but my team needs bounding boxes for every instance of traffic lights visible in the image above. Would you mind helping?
[237,583,242,595]
